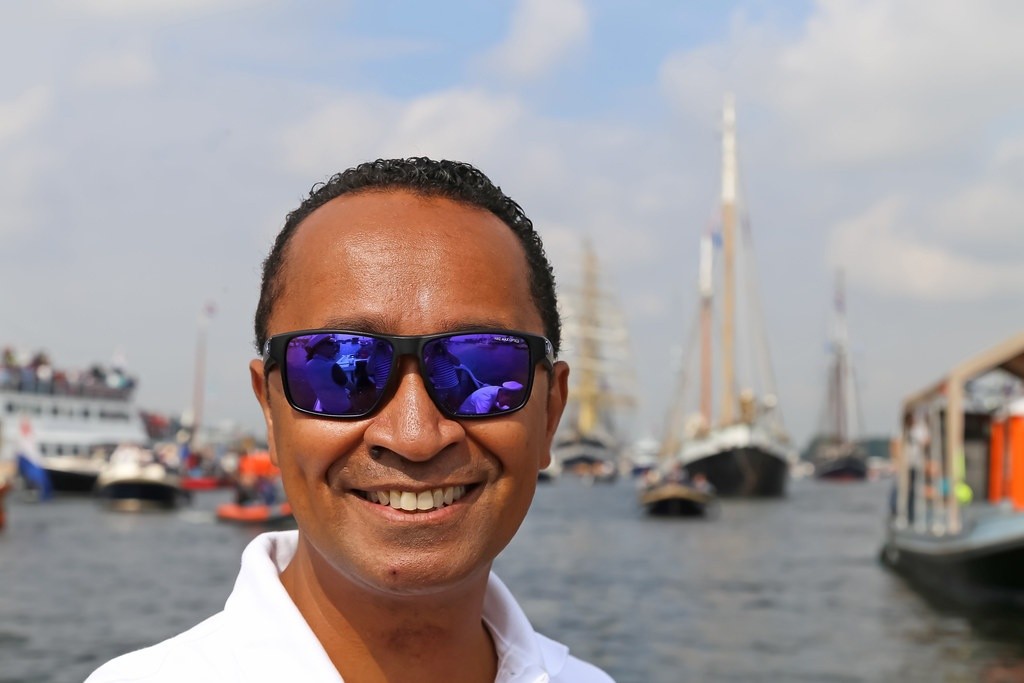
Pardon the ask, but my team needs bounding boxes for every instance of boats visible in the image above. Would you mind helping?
[641,443,708,513]
[0,315,298,525]
[881,339,1023,615]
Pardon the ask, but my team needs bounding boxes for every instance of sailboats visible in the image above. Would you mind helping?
[674,102,795,500]
[806,306,870,481]
[555,226,628,478]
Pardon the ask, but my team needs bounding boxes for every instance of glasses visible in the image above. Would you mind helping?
[262,328,554,419]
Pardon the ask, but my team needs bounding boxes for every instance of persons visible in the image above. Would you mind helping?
[85,156,618,683]
